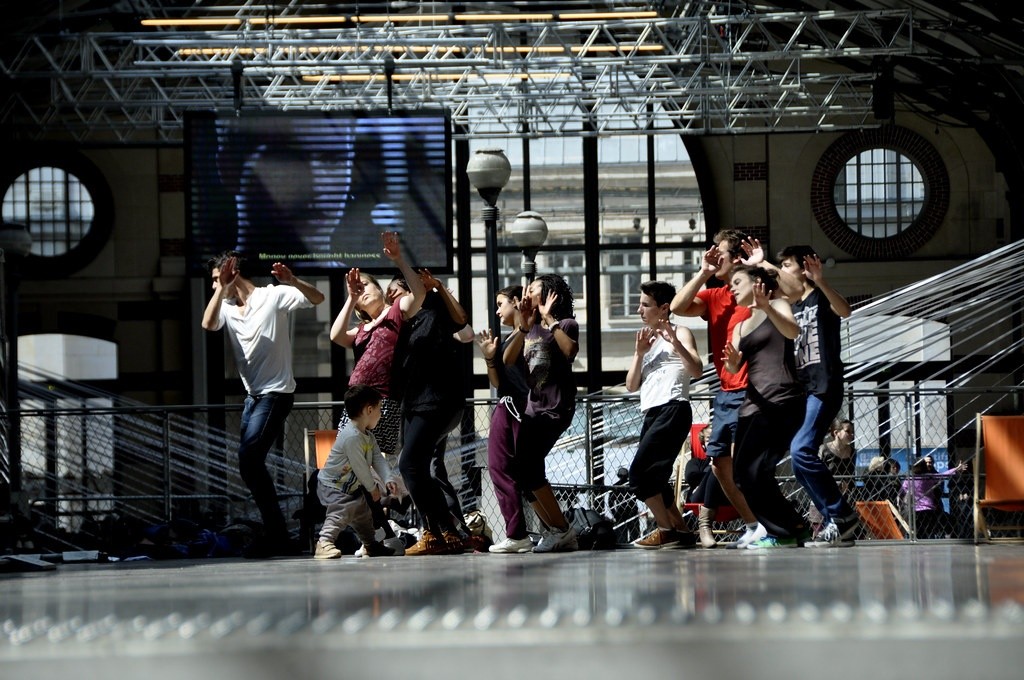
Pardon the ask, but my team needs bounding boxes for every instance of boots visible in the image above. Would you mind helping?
[696,504,718,548]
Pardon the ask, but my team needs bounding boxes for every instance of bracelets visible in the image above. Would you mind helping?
[485,358,495,369]
[548,320,560,333]
[519,326,529,334]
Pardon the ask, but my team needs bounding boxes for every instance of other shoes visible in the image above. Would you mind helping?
[537,533,578,551]
[354,543,367,558]
[804,518,861,547]
[762,527,812,549]
[726,524,768,548]
[383,537,405,556]
[489,535,535,553]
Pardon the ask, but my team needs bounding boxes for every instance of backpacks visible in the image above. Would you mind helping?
[563,507,617,551]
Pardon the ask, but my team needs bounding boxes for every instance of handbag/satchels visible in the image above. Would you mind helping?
[463,509,494,553]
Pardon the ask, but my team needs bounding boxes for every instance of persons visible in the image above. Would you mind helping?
[330,230,426,557]
[776,245,860,545]
[200,251,325,560]
[429,324,475,553]
[386,268,466,554]
[504,274,580,554]
[720,263,807,550]
[313,385,398,558]
[670,229,806,551]
[475,285,533,552]
[1,417,1024,561]
[627,280,702,549]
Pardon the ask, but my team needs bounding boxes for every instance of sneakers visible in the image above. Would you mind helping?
[440,528,466,553]
[633,527,697,549]
[405,528,450,555]
[314,540,341,559]
[365,540,396,557]
[532,524,579,553]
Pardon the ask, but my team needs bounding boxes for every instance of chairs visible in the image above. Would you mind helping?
[973,412,1024,543]
[857,499,913,537]
[303,427,390,558]
